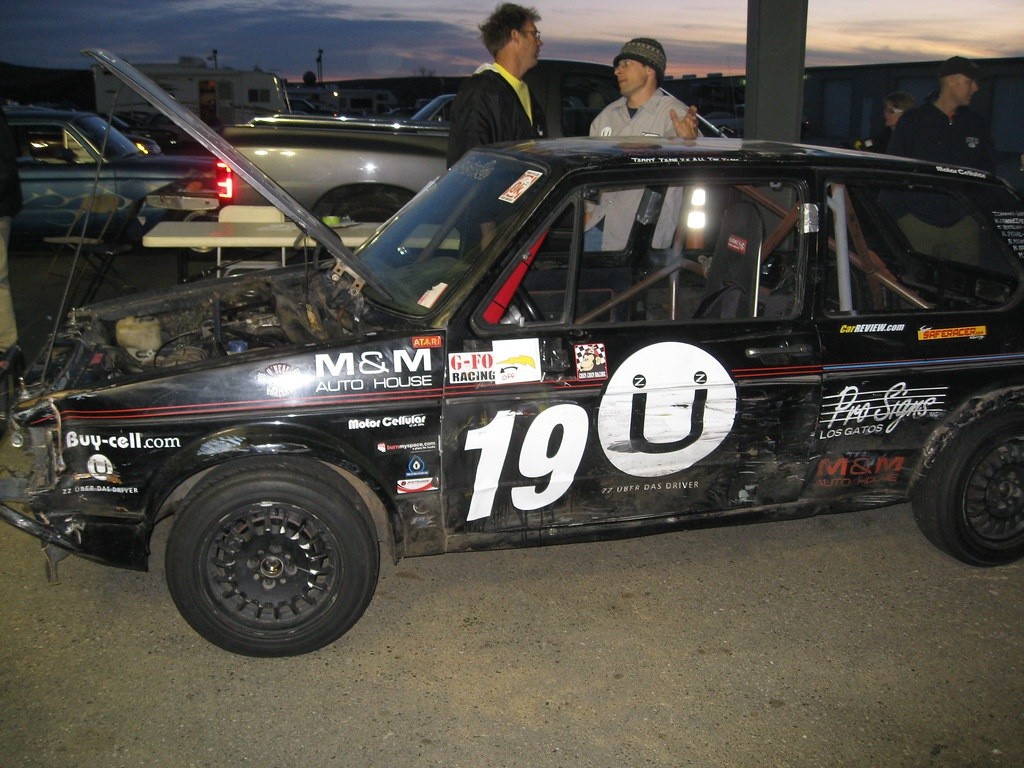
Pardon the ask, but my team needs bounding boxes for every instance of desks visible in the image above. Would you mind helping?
[141,221,460,285]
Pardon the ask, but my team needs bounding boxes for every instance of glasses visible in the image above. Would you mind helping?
[883,106,903,113]
[518,31,540,40]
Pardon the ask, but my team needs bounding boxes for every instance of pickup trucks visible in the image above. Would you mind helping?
[214,60,724,254]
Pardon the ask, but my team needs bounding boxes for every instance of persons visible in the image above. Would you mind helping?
[869,90,917,155]
[879,55,998,308]
[582,37,704,251]
[447,3,549,261]
[0,106,24,370]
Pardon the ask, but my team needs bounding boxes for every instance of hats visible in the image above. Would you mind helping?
[938,56,979,77]
[613,38,666,83]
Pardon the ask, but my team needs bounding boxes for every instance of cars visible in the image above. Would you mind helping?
[0,135,1024,660]
[2,106,227,263]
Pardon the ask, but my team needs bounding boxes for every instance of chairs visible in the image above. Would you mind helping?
[73,197,147,310]
[217,204,287,280]
[44,195,118,290]
[676,202,763,320]
